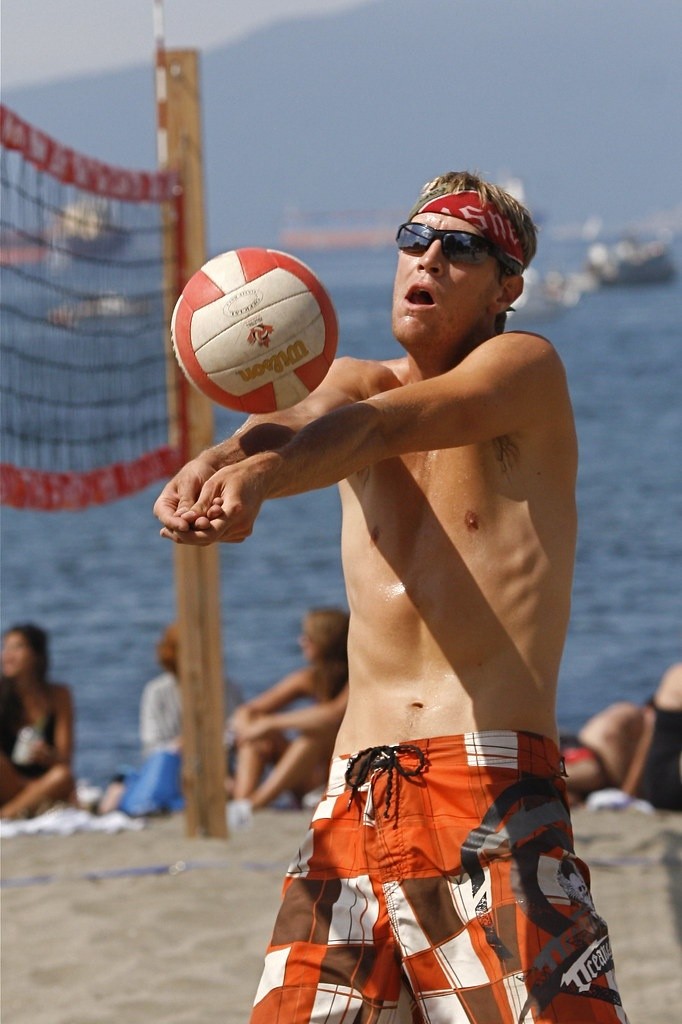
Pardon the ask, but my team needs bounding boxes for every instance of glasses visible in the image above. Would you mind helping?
[397,222,524,280]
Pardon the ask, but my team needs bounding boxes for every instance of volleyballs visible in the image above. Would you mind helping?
[170,245,338,415]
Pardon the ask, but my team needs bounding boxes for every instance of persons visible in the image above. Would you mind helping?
[224,606,350,813]
[95,625,297,814]
[566,661,682,812]
[154,169,630,1024]
[0,624,77,820]
